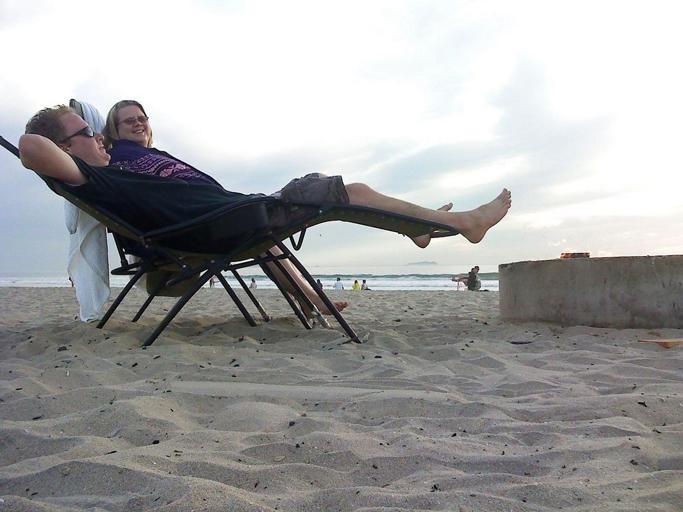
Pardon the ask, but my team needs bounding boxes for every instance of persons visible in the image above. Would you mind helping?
[104,99,350,321]
[316,278,323,290]
[351,280,360,290]
[332,277,344,290]
[210,278,215,288]
[249,277,257,289]
[16,104,511,251]
[359,280,369,290]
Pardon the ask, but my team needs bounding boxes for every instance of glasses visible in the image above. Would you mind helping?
[59,126,93,141]
[117,116,149,125]
